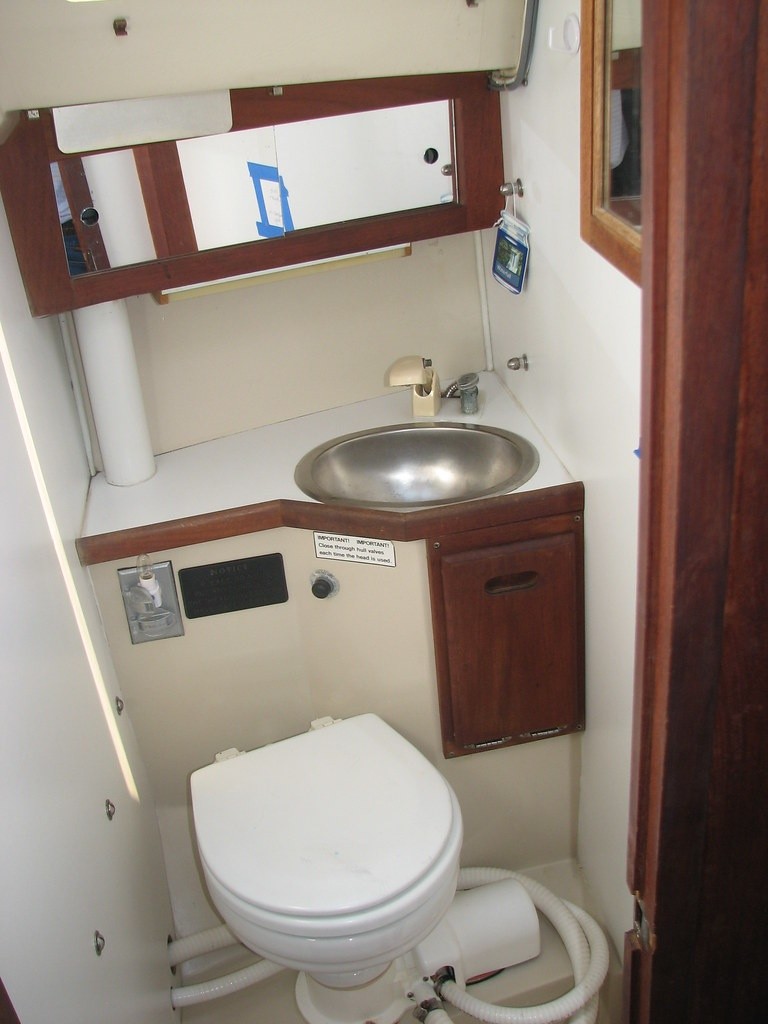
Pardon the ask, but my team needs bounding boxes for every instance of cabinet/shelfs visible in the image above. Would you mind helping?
[423,511,588,758]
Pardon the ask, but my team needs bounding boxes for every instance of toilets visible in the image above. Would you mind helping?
[188,710,462,1024]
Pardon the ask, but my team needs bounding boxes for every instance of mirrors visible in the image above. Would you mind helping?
[49,148,158,277]
[578,2,644,289]
[171,97,457,255]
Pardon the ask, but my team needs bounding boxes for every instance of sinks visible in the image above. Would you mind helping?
[294,420,541,508]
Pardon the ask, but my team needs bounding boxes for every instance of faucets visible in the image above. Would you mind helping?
[445,373,480,414]
